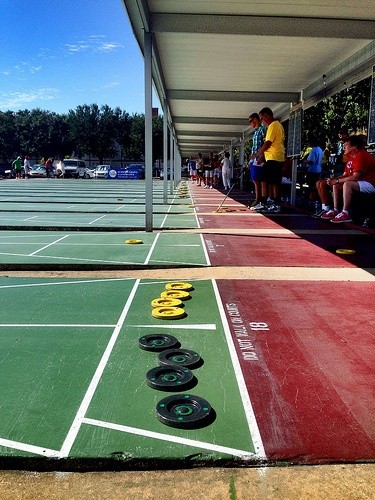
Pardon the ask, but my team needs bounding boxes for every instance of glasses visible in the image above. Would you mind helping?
[249,117,255,125]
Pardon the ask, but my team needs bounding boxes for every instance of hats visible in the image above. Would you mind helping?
[48,158,52,161]
[18,156,21,159]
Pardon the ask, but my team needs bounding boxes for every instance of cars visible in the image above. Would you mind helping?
[29,158,111,179]
[124,164,144,169]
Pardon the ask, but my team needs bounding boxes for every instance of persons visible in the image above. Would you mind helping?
[24,156,31,179]
[220,152,232,190]
[186,151,223,189]
[247,112,268,207]
[12,156,22,179]
[300,131,375,223]
[250,107,285,211]
[45,158,53,177]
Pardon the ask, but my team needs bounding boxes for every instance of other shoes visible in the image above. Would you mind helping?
[314,200,320,205]
[228,186,231,189]
[204,185,209,189]
[196,183,201,186]
[209,186,213,189]
[225,186,228,191]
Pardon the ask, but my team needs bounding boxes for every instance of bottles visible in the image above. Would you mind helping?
[315,201,320,214]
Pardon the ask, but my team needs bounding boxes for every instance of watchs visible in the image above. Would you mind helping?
[336,179,339,184]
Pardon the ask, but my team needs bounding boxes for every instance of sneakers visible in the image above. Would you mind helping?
[321,209,339,219]
[250,202,268,211]
[266,198,274,206]
[263,205,280,213]
[330,212,353,223]
[311,209,327,219]
[247,200,257,209]
